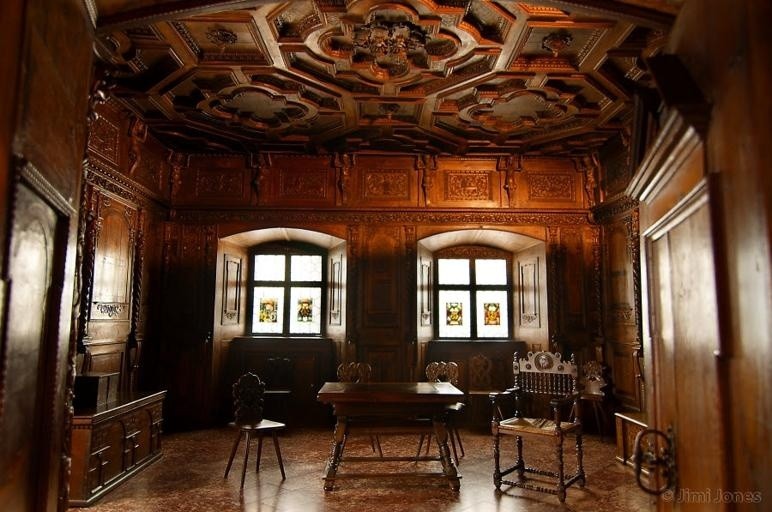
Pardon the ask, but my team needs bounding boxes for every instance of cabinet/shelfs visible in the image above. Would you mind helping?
[68,371,169,508]
[613,410,651,479]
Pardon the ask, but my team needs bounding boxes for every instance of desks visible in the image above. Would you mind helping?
[317,380,466,494]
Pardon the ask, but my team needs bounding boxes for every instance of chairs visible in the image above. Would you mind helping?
[258,358,293,426]
[568,359,610,445]
[223,371,287,491]
[334,361,384,463]
[467,352,505,436]
[413,361,466,468]
[488,350,587,504]
